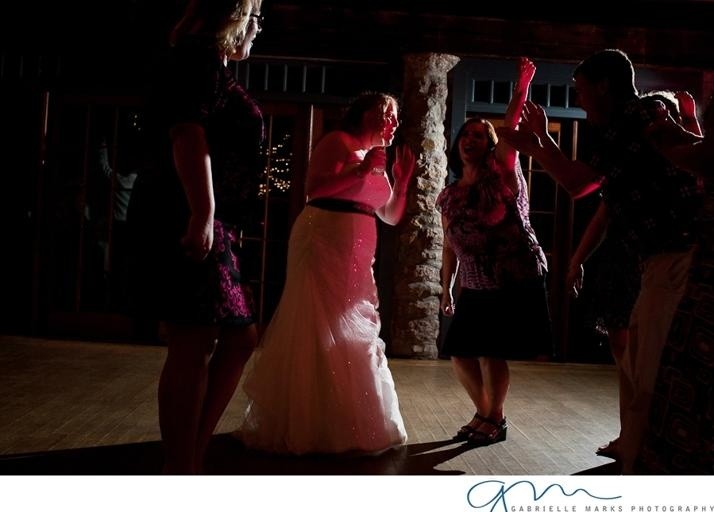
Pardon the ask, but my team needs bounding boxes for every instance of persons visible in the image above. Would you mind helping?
[433,55,550,446]
[494,48,713,475]
[141,1,259,474]
[98,146,140,277]
[232,93,418,457]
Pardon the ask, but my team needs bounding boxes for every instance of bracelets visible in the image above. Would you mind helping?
[352,170,364,179]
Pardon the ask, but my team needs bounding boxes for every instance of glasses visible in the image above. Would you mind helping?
[248,12,266,30]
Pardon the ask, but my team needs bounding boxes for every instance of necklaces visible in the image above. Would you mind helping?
[356,135,370,152]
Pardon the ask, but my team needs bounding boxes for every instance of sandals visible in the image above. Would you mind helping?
[456,413,485,439]
[468,417,508,447]
